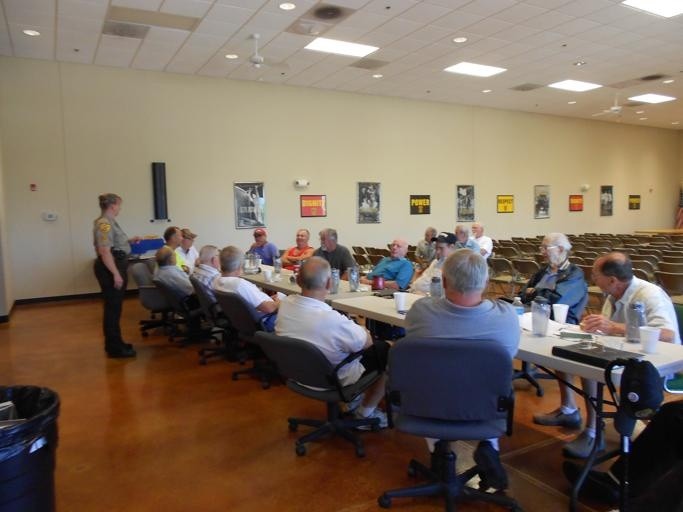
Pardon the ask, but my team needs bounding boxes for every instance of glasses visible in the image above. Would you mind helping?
[537,243,561,252]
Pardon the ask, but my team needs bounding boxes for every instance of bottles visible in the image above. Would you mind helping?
[624,302,647,343]
[580,306,599,332]
[347,266,359,292]
[372,274,384,290]
[531,296,550,337]
[512,297,524,329]
[430,276,441,298]
[330,268,339,294]
[274,255,282,273]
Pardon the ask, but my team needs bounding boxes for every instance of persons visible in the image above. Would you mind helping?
[359,186,370,207]
[532,251,682,459]
[517,232,589,324]
[455,224,480,254]
[414,227,437,262]
[275,257,388,431]
[471,222,493,260]
[361,197,370,208]
[192,245,229,320]
[175,228,199,277]
[212,245,279,333]
[153,247,201,310]
[366,184,378,208]
[93,193,142,358]
[155,227,186,271]
[408,232,459,297]
[311,228,359,281]
[248,185,262,223]
[281,229,314,271]
[359,239,415,290]
[404,248,522,490]
[245,228,280,266]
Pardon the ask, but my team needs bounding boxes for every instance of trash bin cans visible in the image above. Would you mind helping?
[0,386,60,512]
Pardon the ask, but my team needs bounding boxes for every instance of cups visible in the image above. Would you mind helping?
[242,253,263,275]
[639,326,660,353]
[393,293,407,312]
[262,268,272,282]
[553,304,569,324]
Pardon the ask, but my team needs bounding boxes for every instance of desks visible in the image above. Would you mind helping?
[236,256,683,512]
[237,263,380,301]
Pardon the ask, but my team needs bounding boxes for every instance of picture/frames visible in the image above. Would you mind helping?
[533,185,550,218]
[497,195,514,212]
[356,181,382,224]
[569,195,584,211]
[301,195,327,218]
[630,195,641,210]
[601,185,613,217]
[410,195,430,215]
[457,184,475,222]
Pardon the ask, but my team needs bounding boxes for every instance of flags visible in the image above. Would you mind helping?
[675,188,683,229]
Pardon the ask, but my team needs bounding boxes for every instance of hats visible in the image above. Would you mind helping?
[180,229,198,240]
[253,229,267,237]
[430,232,457,244]
[613,358,664,437]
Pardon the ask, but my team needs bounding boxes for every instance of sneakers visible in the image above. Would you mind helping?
[351,408,393,430]
[345,398,364,411]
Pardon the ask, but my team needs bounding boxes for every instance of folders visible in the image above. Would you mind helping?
[552,340,648,372]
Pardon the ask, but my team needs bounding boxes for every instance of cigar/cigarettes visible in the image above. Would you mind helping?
[579,322,587,328]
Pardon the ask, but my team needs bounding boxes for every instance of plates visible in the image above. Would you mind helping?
[396,310,409,315]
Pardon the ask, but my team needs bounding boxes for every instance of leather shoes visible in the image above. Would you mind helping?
[425,451,456,485]
[532,407,582,431]
[560,460,623,505]
[472,439,508,490]
[562,425,606,459]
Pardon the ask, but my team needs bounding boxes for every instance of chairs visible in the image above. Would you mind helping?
[378,332,523,511]
[131,261,278,389]
[256,332,383,458]
